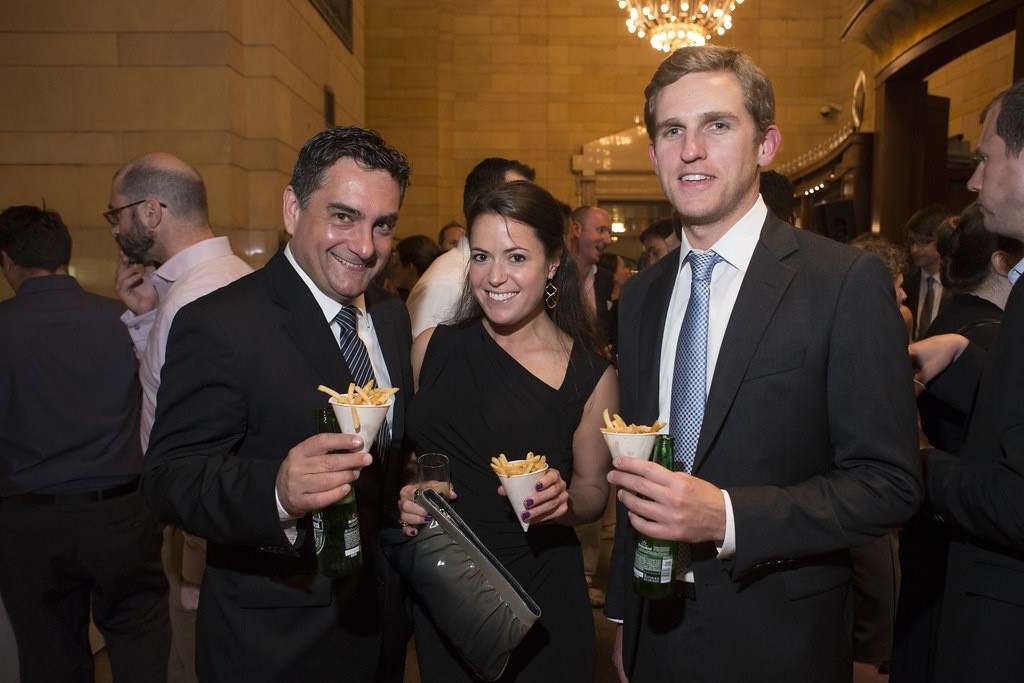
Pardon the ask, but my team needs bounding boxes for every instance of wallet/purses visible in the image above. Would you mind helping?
[403,487,542,683]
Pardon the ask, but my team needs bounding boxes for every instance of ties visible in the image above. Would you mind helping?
[919,276,938,345]
[665,250,724,578]
[333,306,402,467]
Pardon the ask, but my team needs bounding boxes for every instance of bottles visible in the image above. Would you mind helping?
[312,407,363,577]
[631,435,676,600]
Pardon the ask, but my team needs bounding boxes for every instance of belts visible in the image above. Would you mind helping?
[11,475,144,507]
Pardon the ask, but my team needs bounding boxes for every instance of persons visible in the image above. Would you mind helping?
[0,203,172,683]
[141,126,414,683]
[758,172,798,224]
[601,48,917,683]
[858,82,1024,683]
[403,156,540,342]
[388,180,617,683]
[102,152,264,683]
[566,202,684,358]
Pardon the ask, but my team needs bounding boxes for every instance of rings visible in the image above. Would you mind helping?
[414,490,419,501]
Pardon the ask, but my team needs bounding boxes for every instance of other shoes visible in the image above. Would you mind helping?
[583,571,608,608]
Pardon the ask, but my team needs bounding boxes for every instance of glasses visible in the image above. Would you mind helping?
[903,238,935,247]
[104,199,171,226]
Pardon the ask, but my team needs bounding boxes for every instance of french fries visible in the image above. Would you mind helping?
[489,451,548,476]
[599,408,668,433]
[318,379,400,429]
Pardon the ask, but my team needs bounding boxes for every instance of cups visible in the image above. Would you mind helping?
[416,453,451,507]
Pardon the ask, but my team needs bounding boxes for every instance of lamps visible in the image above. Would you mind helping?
[619,0,744,51]
